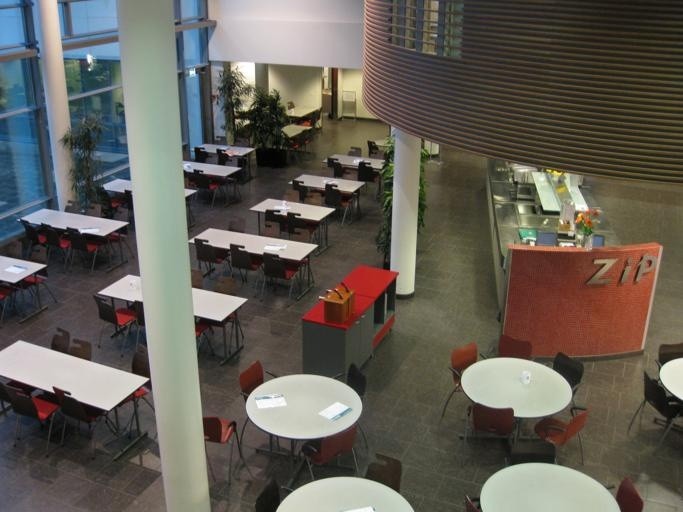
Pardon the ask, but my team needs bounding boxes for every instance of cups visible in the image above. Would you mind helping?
[520,370,531,385]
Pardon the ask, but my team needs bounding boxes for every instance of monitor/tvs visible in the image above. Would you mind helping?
[592,235,604,247]
[537,231,557,247]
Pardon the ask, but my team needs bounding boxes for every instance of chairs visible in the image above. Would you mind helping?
[1,120,415,512]
[439,337,682,512]
